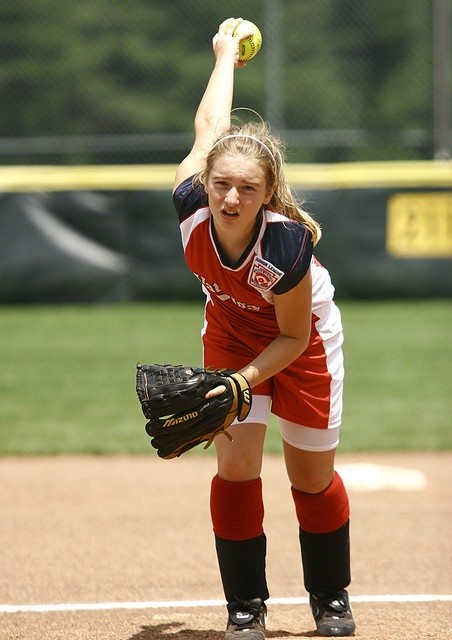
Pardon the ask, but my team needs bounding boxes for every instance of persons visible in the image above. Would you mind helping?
[135,15,358,640]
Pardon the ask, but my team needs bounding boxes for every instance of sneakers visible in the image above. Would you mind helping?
[309,590,355,636]
[225,598,267,640]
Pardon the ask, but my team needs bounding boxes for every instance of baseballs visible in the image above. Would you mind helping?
[232,19,263,61]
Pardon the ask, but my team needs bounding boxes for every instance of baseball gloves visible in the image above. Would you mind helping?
[136,361,252,459]
